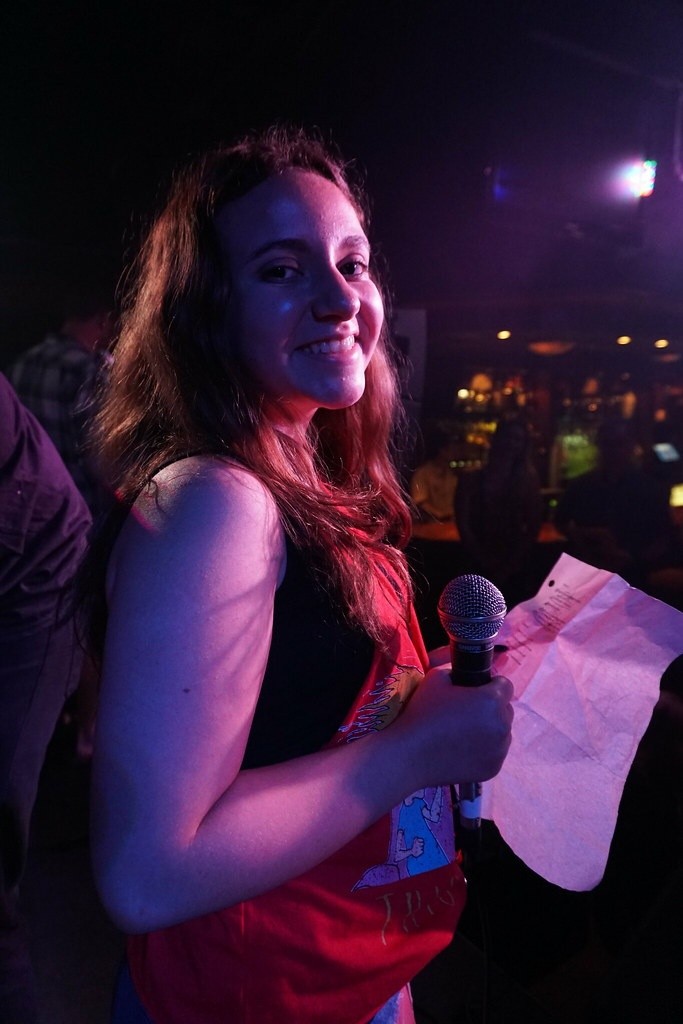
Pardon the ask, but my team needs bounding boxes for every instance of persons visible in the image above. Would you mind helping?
[409,407,669,610]
[0,375,91,897]
[81,130,515,1017]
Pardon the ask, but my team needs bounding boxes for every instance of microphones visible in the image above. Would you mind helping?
[438,574,507,850]
